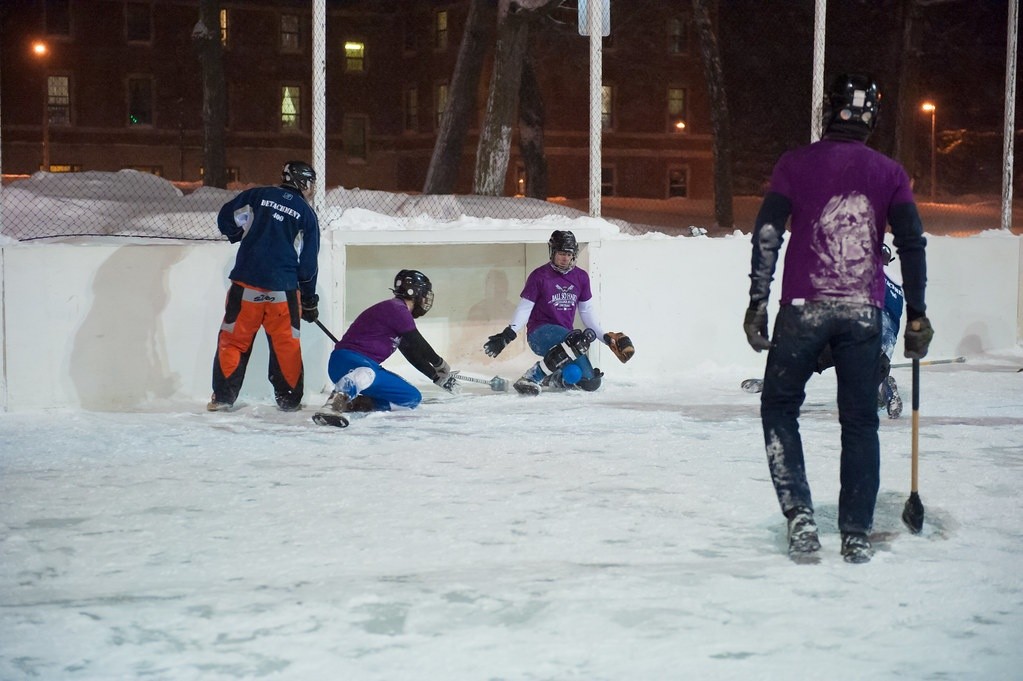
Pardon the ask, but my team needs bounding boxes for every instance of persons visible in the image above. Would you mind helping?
[744,73,934,564]
[206,159,321,412]
[876,243,903,419]
[312,269,463,429]
[483,230,635,397]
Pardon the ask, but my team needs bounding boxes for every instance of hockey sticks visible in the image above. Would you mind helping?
[901,358,924,535]
[889,356,966,368]
[448,372,511,392]
[313,319,338,343]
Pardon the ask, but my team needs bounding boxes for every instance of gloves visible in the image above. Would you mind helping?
[228,226,245,244]
[484,327,518,358]
[301,295,319,322]
[433,375,462,395]
[435,359,451,378]
[904,305,935,360]
[744,276,773,352]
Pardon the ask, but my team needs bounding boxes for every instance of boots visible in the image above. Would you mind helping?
[312,392,349,428]
[346,396,372,412]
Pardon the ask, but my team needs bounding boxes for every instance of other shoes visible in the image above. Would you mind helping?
[207,400,231,410]
[787,515,821,555]
[280,403,302,411]
[513,377,541,396]
[841,533,875,563]
[741,378,765,393]
[883,376,903,418]
[538,374,551,389]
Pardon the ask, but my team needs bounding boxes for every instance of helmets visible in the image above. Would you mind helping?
[280,161,317,191]
[549,230,580,272]
[829,74,882,130]
[394,269,434,318]
[881,243,892,266]
